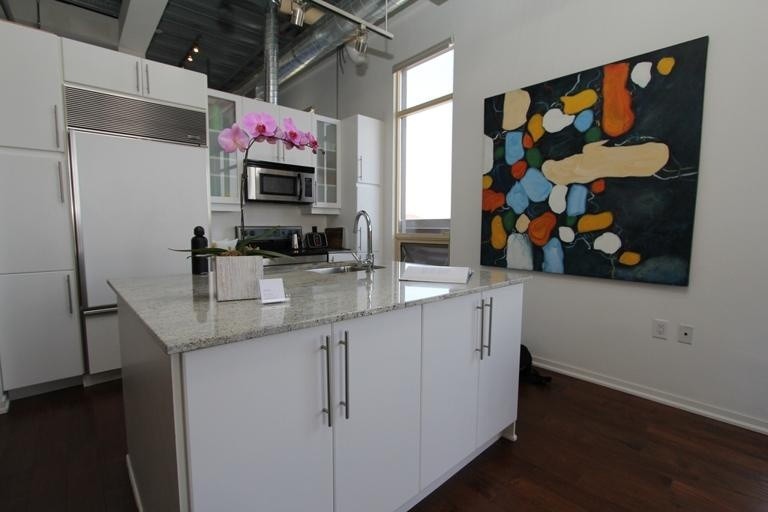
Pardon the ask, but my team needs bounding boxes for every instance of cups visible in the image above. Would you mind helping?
[326,228,343,248]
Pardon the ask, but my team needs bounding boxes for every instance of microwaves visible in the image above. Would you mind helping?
[246,164,316,204]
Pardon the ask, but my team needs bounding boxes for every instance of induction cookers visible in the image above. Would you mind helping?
[252,248,328,258]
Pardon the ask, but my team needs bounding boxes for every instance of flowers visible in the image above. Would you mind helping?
[167,112,324,263]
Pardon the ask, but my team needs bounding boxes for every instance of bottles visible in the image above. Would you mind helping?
[191,226,206,274]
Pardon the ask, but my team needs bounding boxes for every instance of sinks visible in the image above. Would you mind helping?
[308,263,385,275]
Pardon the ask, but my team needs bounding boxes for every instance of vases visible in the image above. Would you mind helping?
[210,254,264,302]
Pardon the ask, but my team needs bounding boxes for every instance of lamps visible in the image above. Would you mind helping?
[289,0,394,66]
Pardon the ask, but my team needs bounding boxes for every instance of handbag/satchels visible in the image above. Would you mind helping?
[520,344,532,378]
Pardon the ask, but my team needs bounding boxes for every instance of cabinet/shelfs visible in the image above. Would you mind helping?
[241,97,314,169]
[302,112,342,217]
[328,114,381,263]
[1,20,88,391]
[207,87,244,211]
[60,35,209,110]
[116,283,523,511]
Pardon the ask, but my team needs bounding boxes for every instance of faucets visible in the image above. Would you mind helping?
[353,210,374,269]
